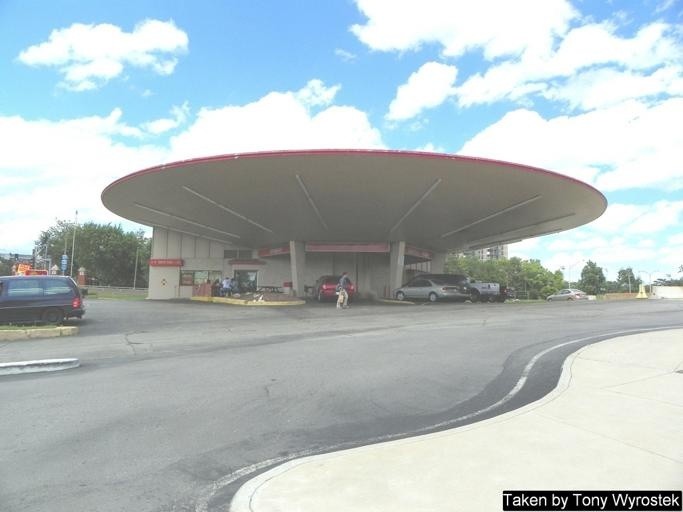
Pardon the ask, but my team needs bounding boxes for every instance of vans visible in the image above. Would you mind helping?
[0,275,87,326]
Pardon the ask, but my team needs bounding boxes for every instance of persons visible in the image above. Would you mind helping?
[337,272,350,308]
[214,278,240,297]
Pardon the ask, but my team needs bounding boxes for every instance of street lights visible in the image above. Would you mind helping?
[70,208,80,279]
[567,257,586,290]
[42,243,48,272]
[628,274,633,293]
[637,269,662,296]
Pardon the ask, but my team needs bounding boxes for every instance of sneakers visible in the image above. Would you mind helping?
[336,305,351,310]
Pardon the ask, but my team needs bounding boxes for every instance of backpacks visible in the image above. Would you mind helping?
[336,280,344,295]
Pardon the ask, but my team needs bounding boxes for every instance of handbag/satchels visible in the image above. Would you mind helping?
[338,294,345,304]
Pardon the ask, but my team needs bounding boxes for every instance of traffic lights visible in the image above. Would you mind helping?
[14,254,18,261]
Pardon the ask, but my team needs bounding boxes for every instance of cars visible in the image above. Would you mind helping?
[392,272,511,304]
[546,288,588,301]
[310,274,355,303]
